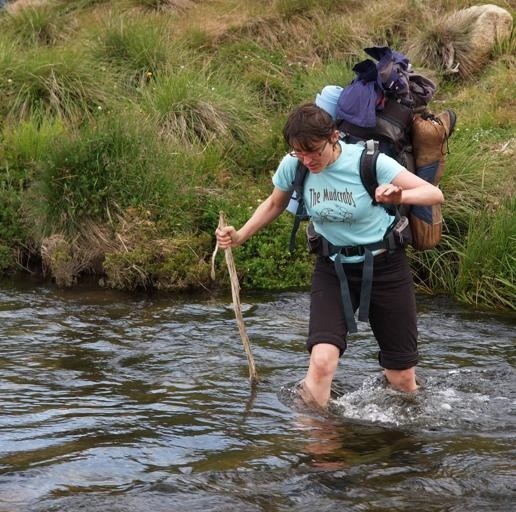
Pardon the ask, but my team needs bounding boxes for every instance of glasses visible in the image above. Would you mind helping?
[289,139,328,158]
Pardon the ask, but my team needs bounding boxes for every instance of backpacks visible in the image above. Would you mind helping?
[306,98,414,258]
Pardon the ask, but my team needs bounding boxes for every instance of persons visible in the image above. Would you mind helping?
[214,102,446,425]
